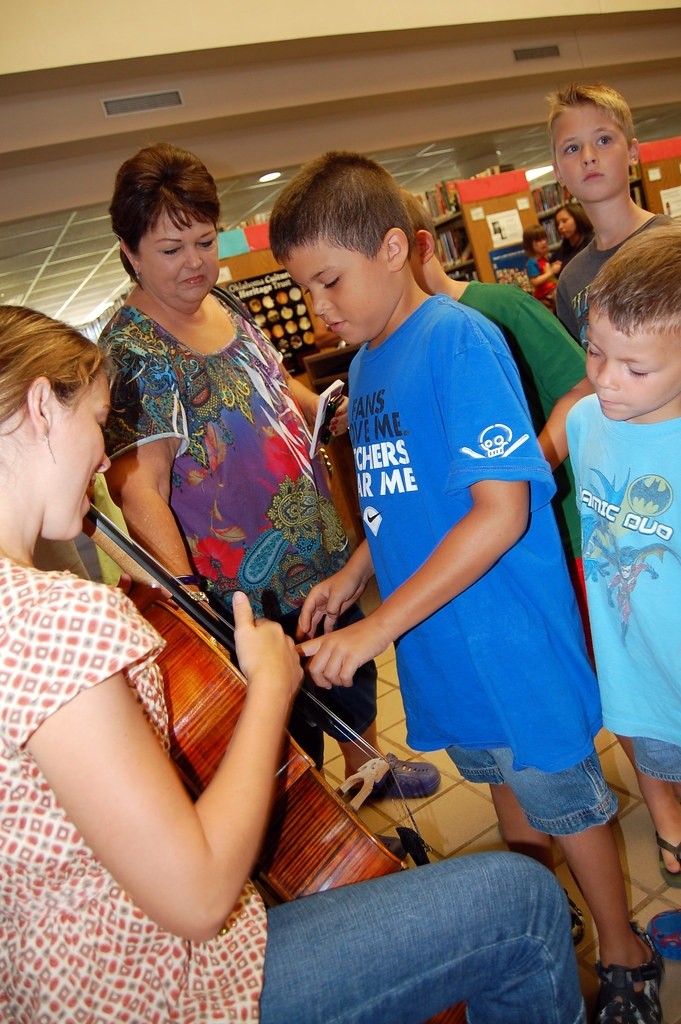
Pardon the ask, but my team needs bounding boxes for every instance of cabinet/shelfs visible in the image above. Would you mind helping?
[532,153,681,251]
[433,191,541,286]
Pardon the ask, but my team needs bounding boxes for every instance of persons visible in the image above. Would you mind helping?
[93,144,440,800]
[565,222,680,888]
[270,150,663,1024]
[522,85,672,350]
[1,304,587,1024]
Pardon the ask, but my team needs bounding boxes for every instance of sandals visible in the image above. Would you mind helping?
[563,887,664,1024]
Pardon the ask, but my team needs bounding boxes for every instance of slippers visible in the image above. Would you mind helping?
[348,751,442,799]
[655,831,681,888]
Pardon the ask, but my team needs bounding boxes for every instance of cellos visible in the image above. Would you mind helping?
[80,500,470,1024]
